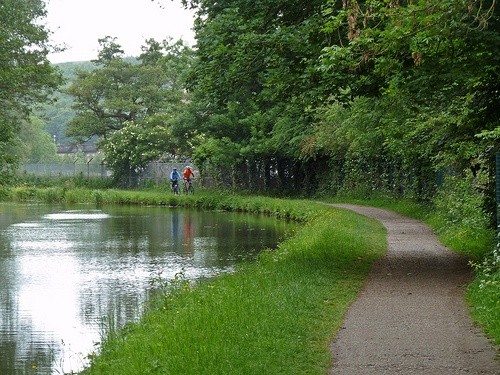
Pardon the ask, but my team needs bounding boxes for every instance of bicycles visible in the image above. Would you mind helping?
[183,177,196,196]
[170,178,181,195]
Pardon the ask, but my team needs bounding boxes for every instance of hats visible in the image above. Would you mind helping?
[186,166,192,169]
[173,168,177,171]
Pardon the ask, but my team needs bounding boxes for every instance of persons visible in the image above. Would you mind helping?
[170,168,181,194]
[182,166,195,194]
